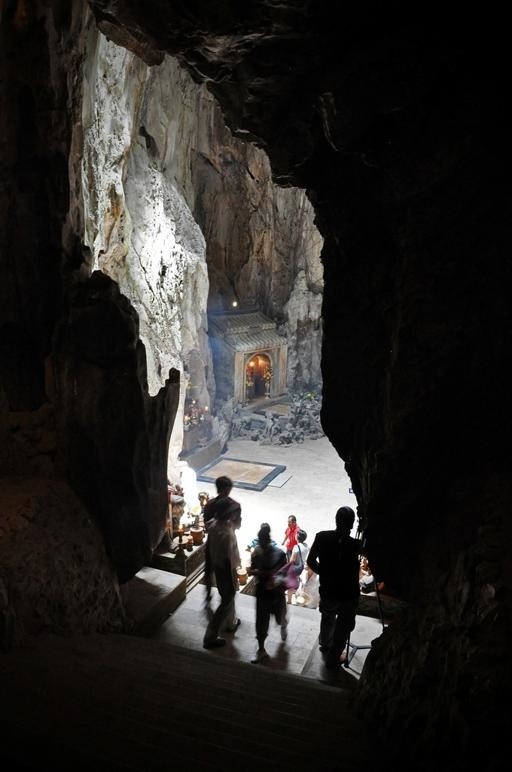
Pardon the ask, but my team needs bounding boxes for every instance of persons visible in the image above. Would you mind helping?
[198,492,213,513]
[288,530,310,605]
[203,499,241,649]
[250,522,278,554]
[281,515,301,563]
[306,505,368,669]
[250,528,288,664]
[205,476,242,609]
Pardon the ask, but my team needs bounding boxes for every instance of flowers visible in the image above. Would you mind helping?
[246,379,255,390]
[260,365,274,382]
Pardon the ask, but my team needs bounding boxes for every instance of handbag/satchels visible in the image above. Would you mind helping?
[287,559,304,576]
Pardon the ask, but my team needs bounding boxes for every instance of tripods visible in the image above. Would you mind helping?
[344,531,386,667]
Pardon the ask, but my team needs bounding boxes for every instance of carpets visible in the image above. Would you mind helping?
[197,456,292,491]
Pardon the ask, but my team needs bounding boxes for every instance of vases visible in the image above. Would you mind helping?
[264,382,270,400]
[245,389,251,404]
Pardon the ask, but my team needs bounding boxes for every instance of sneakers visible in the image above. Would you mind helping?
[279,624,288,641]
[250,649,268,664]
[203,635,226,649]
[228,619,241,635]
